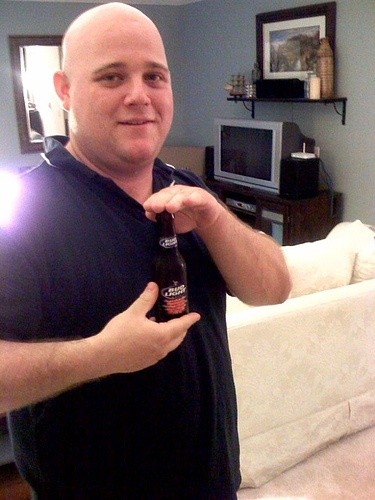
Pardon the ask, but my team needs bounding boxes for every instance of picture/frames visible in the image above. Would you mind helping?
[256,2,336,99]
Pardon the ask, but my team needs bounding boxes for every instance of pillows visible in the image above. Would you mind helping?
[279,245,355,297]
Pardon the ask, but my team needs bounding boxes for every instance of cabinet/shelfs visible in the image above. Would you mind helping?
[201,178,344,246]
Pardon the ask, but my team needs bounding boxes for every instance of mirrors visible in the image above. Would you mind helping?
[8,33,67,154]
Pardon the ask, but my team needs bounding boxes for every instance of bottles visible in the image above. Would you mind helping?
[153,209,189,322]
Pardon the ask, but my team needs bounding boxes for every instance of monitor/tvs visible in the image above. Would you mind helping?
[213,119,315,196]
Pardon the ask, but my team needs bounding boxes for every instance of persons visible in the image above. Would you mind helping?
[0,0,294,500]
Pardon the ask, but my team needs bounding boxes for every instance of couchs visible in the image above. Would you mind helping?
[225,220,375,489]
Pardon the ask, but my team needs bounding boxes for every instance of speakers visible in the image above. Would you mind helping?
[280,159,319,199]
[204,146,215,180]
